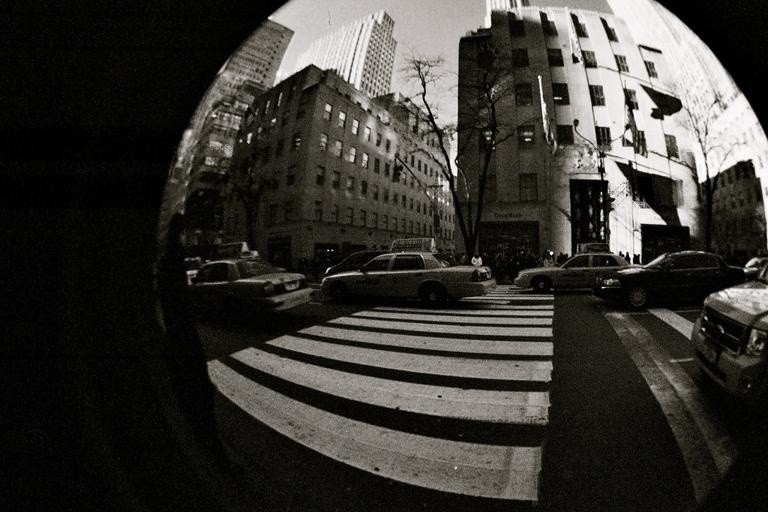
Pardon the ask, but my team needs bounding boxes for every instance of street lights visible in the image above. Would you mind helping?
[573,118,632,181]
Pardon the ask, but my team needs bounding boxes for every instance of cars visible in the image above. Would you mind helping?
[513,253,630,294]
[318,236,498,308]
[592,249,760,312]
[190,255,315,319]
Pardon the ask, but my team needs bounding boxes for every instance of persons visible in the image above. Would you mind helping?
[431,242,642,283]
